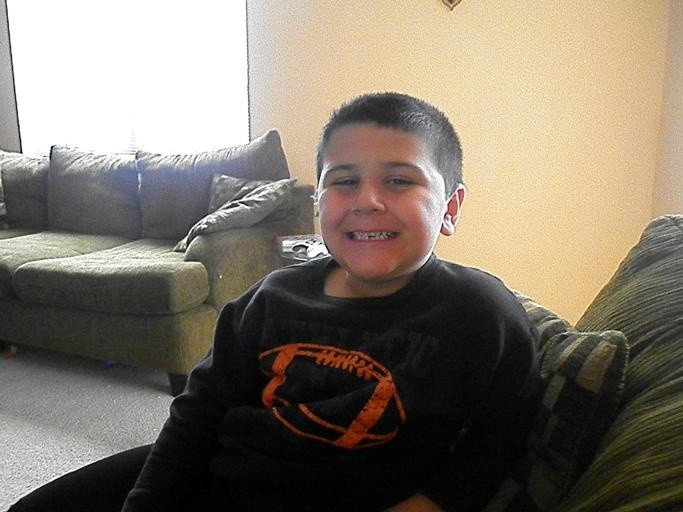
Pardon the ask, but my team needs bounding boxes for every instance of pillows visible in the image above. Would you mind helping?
[0,130,297,253]
[505,287,628,509]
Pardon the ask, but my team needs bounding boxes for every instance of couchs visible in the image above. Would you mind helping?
[0,127,318,398]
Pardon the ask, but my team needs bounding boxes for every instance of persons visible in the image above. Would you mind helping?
[8,92,544,511]
